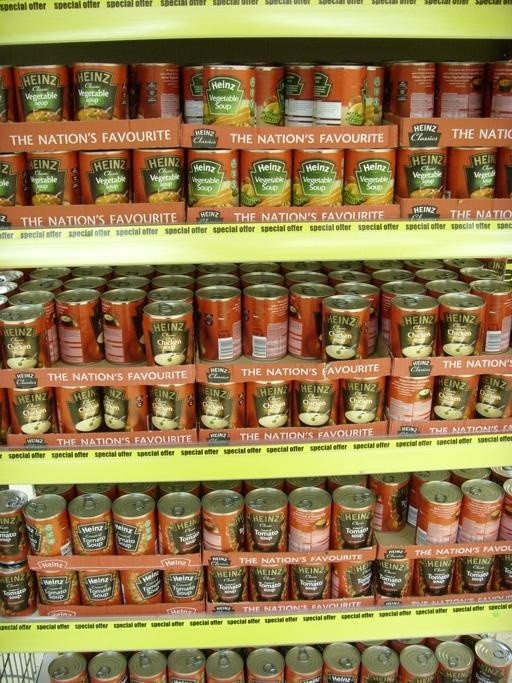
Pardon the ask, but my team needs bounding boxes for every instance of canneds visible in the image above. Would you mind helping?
[0,60,512,209]
[0,466,512,617]
[0,258,512,436]
[48,634,512,683]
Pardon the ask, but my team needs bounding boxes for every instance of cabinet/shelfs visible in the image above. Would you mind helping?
[0,40,512,683]
